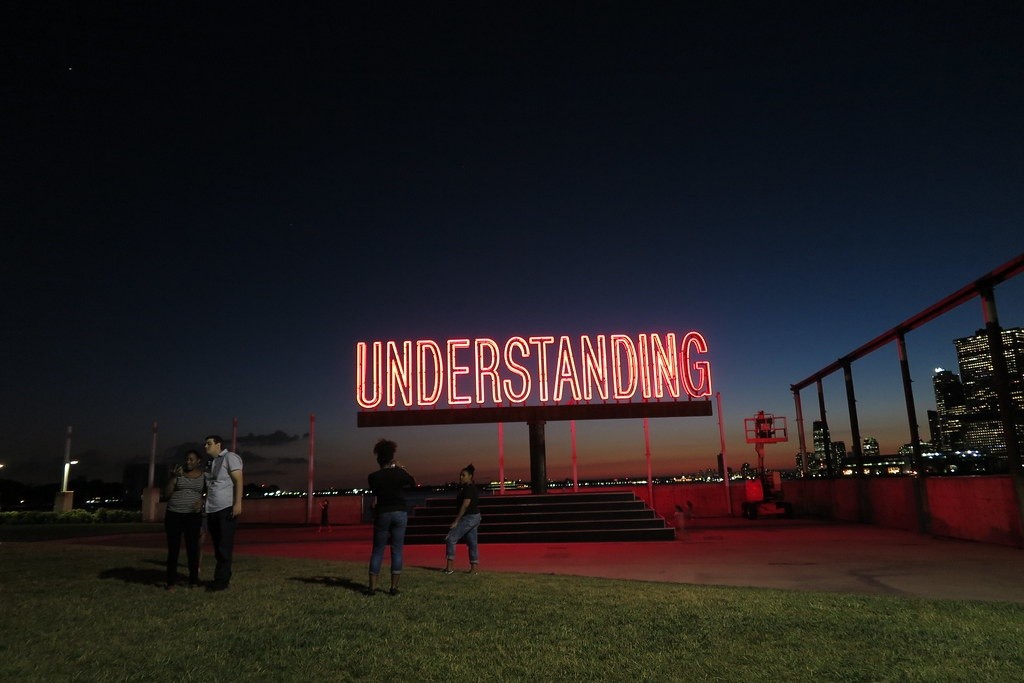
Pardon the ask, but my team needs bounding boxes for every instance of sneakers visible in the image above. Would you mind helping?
[189,581,198,589]
[164,582,176,591]
[441,567,454,575]
[463,568,480,576]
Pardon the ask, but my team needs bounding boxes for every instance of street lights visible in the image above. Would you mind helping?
[59,424,79,492]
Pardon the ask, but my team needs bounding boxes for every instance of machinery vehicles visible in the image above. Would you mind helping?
[738,409,794,521]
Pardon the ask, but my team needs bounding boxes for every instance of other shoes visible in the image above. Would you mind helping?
[363,589,376,596]
[390,586,399,596]
[318,530,321,532]
[207,581,230,590]
[328,530,332,532]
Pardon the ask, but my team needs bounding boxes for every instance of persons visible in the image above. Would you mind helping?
[318,499,332,533]
[163,449,206,593]
[368,440,415,597]
[443,464,480,574]
[202,435,242,592]
